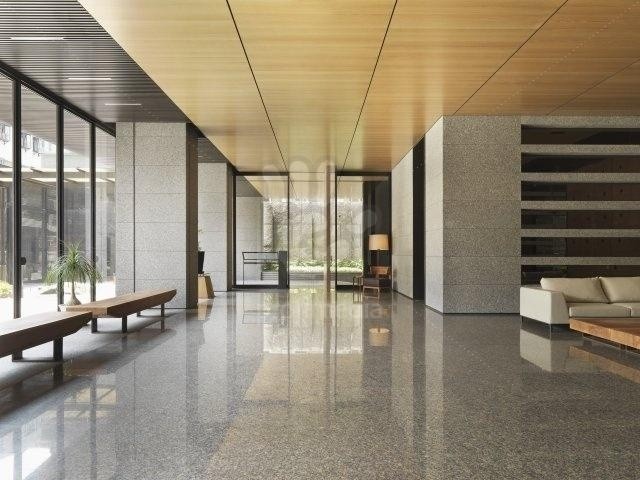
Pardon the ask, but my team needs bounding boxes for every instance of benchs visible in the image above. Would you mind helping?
[1,309,91,361]
[67,289,177,332]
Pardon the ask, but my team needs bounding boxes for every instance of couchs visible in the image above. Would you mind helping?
[515,327,587,376]
[519,284,640,338]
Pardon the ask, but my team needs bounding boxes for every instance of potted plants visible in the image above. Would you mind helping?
[44,232,104,314]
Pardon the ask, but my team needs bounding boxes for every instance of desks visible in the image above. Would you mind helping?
[565,344,640,383]
[569,315,640,350]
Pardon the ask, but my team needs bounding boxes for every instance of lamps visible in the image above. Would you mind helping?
[368,235,390,279]
[369,302,389,347]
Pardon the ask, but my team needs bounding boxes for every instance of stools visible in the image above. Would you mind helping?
[353,276,365,287]
[362,277,391,292]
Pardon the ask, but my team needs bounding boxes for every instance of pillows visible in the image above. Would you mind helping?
[541,277,609,303]
[600,274,640,303]
